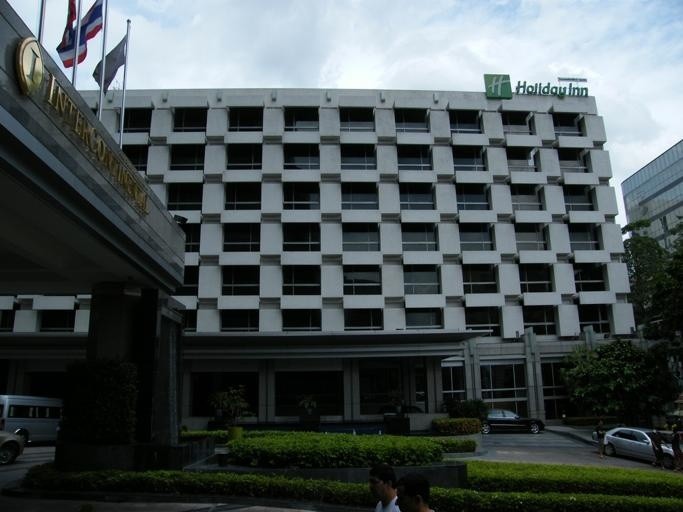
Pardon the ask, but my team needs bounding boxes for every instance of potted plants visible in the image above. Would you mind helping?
[298,394,320,432]
[212,384,250,439]
[383,390,410,434]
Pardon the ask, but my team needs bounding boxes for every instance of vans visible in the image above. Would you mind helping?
[0,394,62,447]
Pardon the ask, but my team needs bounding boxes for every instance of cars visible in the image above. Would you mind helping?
[591,427,683,471]
[0,430,26,466]
[478,408,544,434]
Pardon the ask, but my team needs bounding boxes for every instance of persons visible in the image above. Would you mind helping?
[394,473,435,512]
[596,420,606,458]
[650,427,669,469]
[369,464,401,512]
[670,424,683,472]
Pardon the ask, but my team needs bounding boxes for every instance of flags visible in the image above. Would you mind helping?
[62,0,76,48]
[93,34,127,95]
[56,0,102,68]
